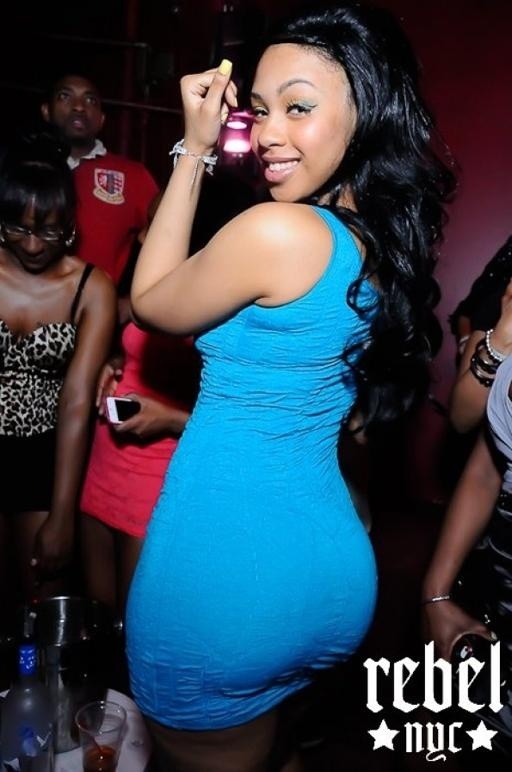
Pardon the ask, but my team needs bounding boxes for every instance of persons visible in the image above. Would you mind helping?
[0,7,511,771]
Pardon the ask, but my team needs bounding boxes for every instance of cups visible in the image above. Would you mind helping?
[76,702,127,772]
[19,594,120,753]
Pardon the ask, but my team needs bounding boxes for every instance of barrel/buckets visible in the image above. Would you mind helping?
[1,596,122,755]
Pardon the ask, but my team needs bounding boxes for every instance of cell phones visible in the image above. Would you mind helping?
[106,396,140,423]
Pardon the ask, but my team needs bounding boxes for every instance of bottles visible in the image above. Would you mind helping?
[2,638,55,772]
[450,634,509,695]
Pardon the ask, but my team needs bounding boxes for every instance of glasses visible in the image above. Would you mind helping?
[2,221,77,248]
